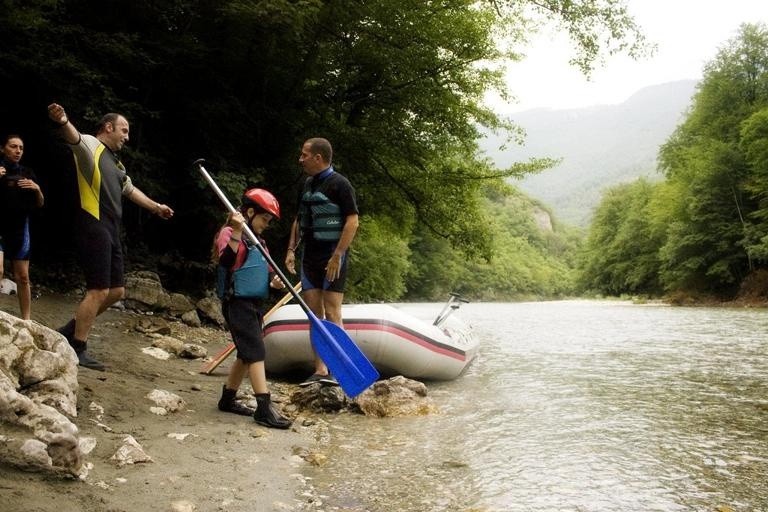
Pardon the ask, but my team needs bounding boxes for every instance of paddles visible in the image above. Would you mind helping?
[193,159,380,398]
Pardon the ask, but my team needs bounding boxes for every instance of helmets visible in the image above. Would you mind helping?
[241,188,280,219]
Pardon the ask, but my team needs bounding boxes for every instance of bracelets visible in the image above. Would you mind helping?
[59,119,70,126]
[229,236,241,243]
[151,202,161,214]
[286,246,295,252]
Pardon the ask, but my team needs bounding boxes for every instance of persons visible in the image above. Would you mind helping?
[286,137,360,388]
[205,189,292,428]
[0,129,47,321]
[47,102,175,372]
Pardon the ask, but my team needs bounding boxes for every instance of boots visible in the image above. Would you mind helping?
[253,393,292,428]
[70,336,104,370]
[218,384,255,416]
[56,318,76,343]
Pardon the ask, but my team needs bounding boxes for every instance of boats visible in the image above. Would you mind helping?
[262,305,477,382]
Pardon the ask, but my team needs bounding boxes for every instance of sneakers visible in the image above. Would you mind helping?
[319,374,339,387]
[299,373,330,388]
